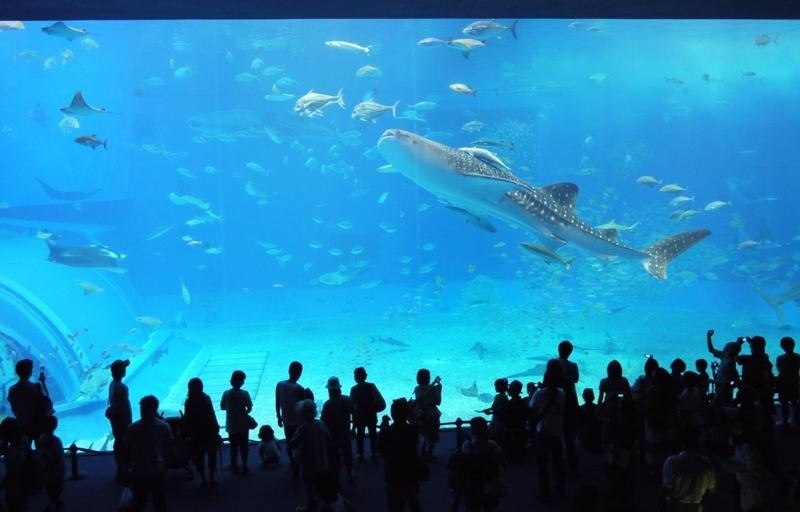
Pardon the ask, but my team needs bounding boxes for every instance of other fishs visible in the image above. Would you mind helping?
[0,18,800,413]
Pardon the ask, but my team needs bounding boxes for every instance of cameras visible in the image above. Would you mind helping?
[740,337,748,342]
[713,362,718,367]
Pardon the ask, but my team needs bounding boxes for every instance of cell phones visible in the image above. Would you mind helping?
[435,376,440,383]
[39,366,45,380]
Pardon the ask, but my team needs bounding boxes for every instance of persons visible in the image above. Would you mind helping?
[1,331,800,512]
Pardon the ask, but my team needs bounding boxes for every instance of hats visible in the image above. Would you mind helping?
[294,399,317,418]
[111,360,129,368]
[324,376,341,390]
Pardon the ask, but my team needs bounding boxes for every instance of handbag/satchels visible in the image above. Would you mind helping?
[105,407,119,419]
